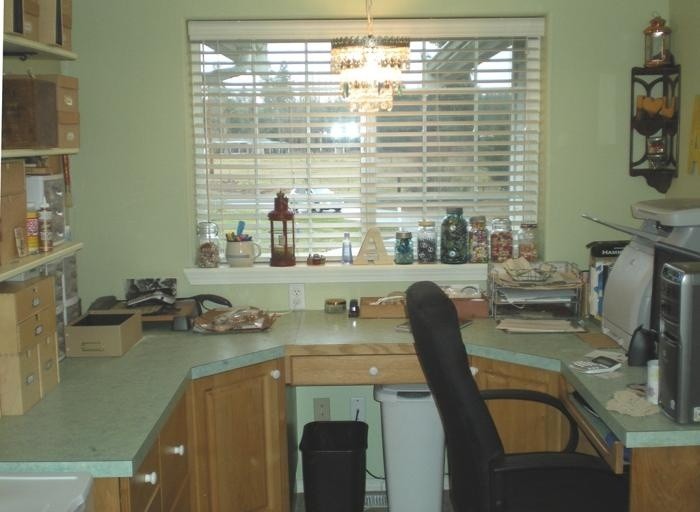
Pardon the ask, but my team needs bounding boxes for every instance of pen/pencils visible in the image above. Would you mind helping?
[226,221,253,241]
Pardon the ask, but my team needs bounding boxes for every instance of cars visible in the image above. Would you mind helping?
[285,186,344,213]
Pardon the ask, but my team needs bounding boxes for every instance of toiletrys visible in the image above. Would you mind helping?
[324,298,347,313]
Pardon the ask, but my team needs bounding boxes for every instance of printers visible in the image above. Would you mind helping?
[580,197,700,353]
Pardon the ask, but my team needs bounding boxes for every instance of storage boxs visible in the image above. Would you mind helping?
[2,158,26,267]
[64,309,143,358]
[3,72,80,150]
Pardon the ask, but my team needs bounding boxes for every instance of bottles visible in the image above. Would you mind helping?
[38,204,53,251]
[439,206,467,263]
[491,218,513,262]
[26,201,38,254]
[194,223,219,267]
[469,215,490,262]
[517,223,541,265]
[394,232,414,265]
[417,220,437,262]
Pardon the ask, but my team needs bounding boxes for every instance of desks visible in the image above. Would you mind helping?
[0,303,698,512]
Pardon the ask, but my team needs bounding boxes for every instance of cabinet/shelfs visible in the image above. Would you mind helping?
[181,350,293,512]
[88,385,199,510]
[628,65,682,193]
[0,0,85,417]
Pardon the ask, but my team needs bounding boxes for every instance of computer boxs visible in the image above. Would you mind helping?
[658,261,700,425]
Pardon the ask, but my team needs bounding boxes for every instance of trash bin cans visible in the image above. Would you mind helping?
[299,420,369,512]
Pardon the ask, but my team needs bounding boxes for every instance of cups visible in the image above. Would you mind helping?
[226,240,261,266]
[645,360,660,405]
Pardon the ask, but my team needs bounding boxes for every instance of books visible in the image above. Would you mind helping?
[393,317,475,333]
[499,294,573,302]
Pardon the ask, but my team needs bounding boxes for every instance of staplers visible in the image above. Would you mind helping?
[349,299,359,318]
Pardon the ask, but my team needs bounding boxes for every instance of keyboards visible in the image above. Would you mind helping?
[572,391,600,419]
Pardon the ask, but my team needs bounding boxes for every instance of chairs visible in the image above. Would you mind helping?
[406,282,626,510]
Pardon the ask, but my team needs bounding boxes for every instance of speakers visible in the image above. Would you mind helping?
[628,326,654,366]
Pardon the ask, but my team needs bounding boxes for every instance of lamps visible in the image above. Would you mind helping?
[328,0,412,115]
[642,14,672,67]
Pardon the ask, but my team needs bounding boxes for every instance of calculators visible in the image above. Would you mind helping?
[569,355,623,374]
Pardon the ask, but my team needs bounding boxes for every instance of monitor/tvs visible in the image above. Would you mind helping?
[648,241,700,343]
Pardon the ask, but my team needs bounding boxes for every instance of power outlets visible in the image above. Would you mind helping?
[349,397,365,422]
[286,283,306,310]
